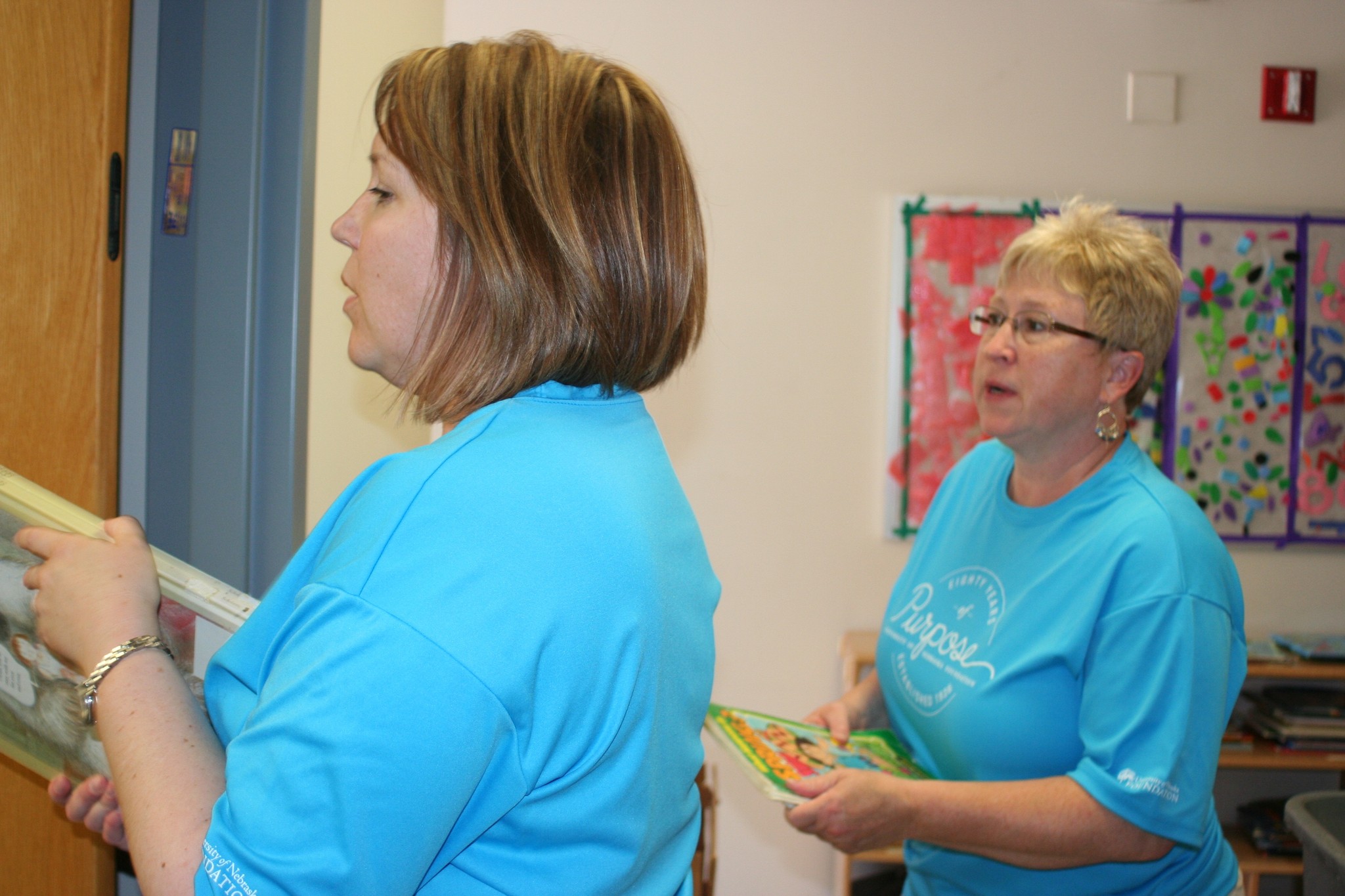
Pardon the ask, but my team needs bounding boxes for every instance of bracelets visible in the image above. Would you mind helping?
[78,632,177,728]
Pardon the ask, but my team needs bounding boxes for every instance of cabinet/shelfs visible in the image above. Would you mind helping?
[832,629,1345,896]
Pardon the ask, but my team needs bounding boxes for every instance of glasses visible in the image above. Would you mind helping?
[968,305,1129,352]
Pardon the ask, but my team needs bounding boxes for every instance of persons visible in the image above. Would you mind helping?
[14,29,724,891]
[780,197,1255,896]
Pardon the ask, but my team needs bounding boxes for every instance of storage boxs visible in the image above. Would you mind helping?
[1283,791,1345,896]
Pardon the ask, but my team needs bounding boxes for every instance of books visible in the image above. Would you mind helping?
[1239,697,1345,753]
[0,465,263,788]
[702,702,938,806]
